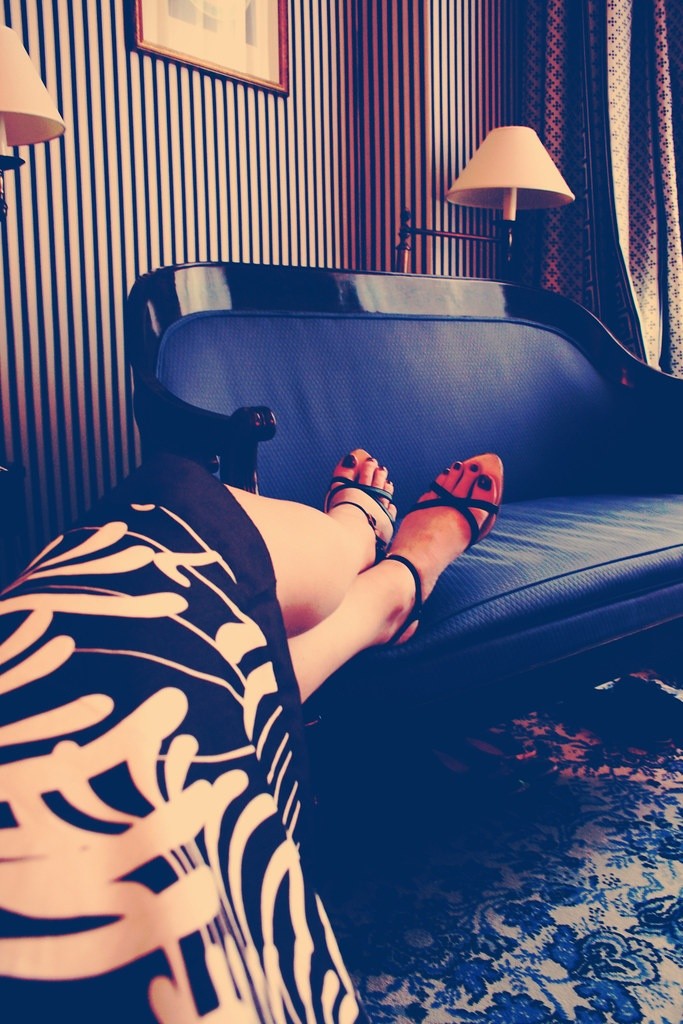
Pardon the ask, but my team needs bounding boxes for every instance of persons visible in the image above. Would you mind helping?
[0,443,505,1024]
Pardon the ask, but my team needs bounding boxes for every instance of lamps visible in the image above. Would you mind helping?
[0,24,68,222]
[396,126,575,274]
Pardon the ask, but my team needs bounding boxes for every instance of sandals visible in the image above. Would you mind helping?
[372,452,504,651]
[323,447,399,566]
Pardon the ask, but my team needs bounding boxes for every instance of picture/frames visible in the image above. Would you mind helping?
[123,0,290,99]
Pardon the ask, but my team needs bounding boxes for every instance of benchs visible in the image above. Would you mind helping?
[123,261,683,744]
